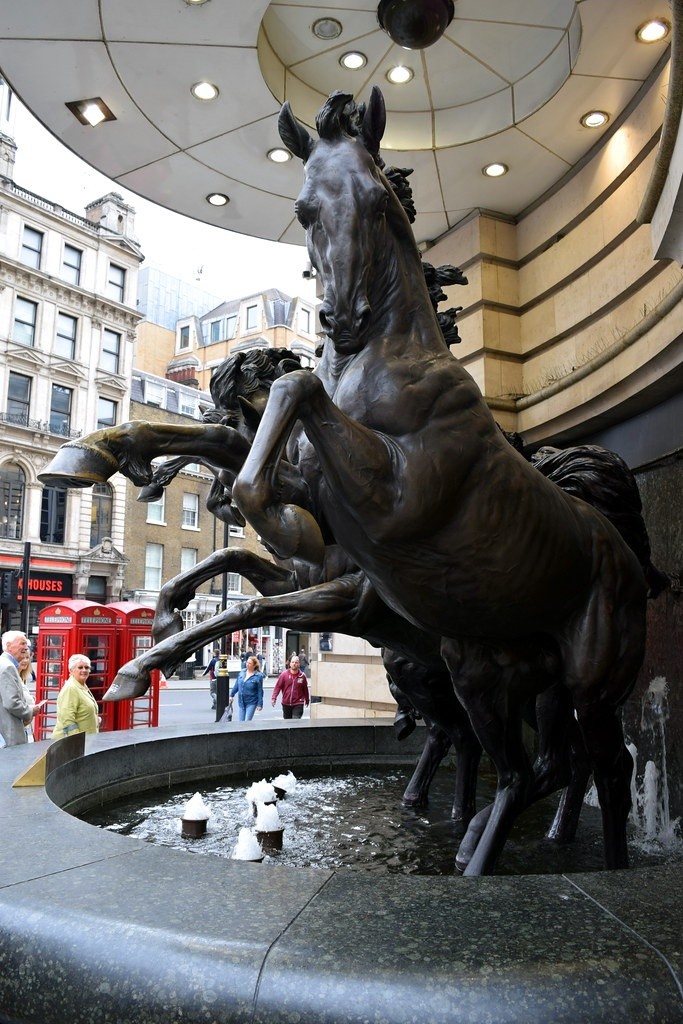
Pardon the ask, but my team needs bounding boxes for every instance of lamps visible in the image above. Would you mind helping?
[81,103,105,127]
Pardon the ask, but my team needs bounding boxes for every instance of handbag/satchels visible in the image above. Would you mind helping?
[219,701,233,722]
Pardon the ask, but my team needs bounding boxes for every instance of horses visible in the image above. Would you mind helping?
[38,85,664,876]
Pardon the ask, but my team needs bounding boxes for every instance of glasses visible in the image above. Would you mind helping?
[79,666,89,669]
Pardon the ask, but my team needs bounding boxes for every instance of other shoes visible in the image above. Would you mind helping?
[211,704,217,709]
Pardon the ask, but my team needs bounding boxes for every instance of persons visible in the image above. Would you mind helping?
[319,631,334,652]
[0,630,42,752]
[240,647,262,670]
[271,656,310,719]
[228,651,264,721]
[52,654,102,739]
[202,648,219,710]
[287,648,309,672]
